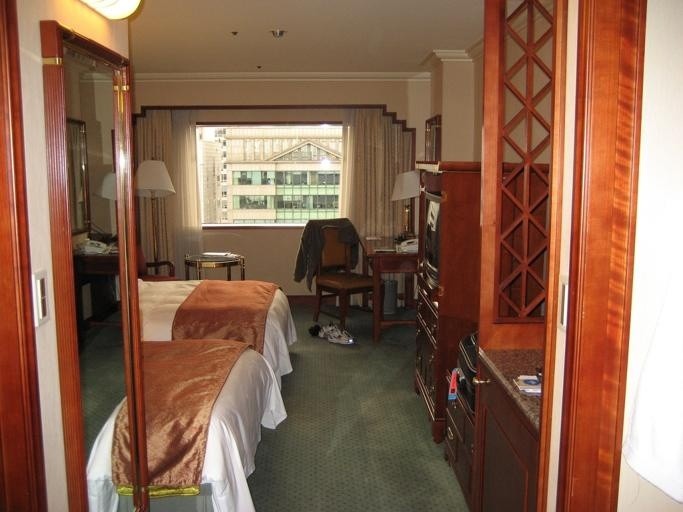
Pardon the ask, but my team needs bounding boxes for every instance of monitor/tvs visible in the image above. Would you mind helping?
[422,191,442,289]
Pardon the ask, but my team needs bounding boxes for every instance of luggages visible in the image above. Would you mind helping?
[451,331,479,411]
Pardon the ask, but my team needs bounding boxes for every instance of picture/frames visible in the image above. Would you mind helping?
[423,114,441,162]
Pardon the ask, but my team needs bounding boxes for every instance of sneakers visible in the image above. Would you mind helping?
[318,322,354,345]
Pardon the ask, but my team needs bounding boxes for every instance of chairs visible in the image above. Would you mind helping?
[309,218,385,332]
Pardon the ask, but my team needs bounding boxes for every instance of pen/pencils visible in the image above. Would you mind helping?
[376,251,396,253]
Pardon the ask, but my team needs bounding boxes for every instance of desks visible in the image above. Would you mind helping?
[72,243,120,340]
[359,235,419,344]
[183,253,245,281]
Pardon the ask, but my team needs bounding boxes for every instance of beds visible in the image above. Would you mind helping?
[84,396,136,512]
[136,278,297,390]
[139,339,287,512]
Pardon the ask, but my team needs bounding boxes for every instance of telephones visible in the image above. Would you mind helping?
[400,238,419,254]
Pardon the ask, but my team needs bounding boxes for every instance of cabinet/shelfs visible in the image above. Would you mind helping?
[469,350,540,512]
[414,159,550,444]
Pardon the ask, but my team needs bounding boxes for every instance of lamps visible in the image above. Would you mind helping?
[101,173,117,202]
[391,170,420,240]
[134,160,176,277]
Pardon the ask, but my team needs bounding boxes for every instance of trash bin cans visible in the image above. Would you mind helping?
[383,280,398,314]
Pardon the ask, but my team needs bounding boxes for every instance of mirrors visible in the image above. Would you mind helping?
[65,117,90,236]
[41,20,148,512]
